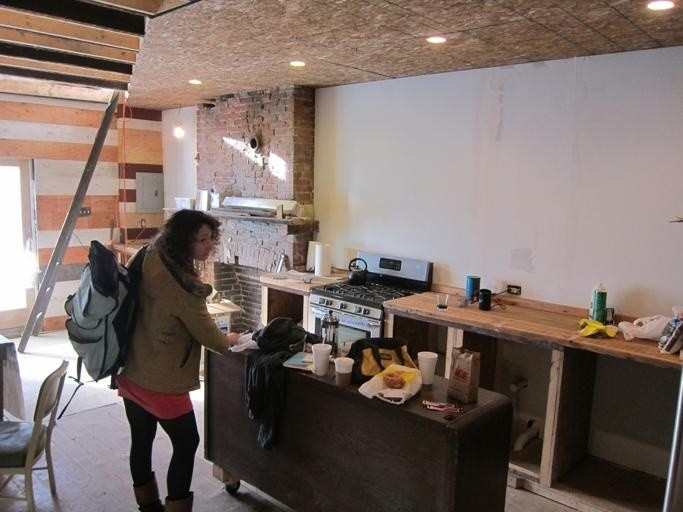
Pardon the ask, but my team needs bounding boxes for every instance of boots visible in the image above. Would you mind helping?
[165,492,194,512]
[133,471,164,512]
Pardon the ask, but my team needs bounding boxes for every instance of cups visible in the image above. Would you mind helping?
[417,351,438,385]
[605,305,616,325]
[479,289,491,312]
[333,356,354,387]
[435,293,449,312]
[310,343,332,376]
[465,276,480,304]
[195,189,206,212]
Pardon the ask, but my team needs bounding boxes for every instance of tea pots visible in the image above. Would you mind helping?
[347,258,369,286]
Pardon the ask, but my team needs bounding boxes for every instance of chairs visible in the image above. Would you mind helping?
[0,358,69,512]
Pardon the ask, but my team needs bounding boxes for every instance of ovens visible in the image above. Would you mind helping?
[309,303,385,354]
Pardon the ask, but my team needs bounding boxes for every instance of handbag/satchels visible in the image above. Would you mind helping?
[346,338,424,405]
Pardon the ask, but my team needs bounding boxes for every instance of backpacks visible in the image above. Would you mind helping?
[65,240,150,382]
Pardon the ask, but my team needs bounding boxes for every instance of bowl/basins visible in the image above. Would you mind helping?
[174,197,195,210]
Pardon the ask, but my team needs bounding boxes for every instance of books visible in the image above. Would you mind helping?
[282,352,314,370]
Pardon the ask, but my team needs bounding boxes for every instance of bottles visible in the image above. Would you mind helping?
[593,282,607,326]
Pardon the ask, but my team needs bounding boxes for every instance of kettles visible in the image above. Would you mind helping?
[318,310,338,356]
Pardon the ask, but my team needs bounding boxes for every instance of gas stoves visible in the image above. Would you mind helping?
[309,276,414,309]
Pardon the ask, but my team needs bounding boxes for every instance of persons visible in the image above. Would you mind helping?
[115,209,239,512]
